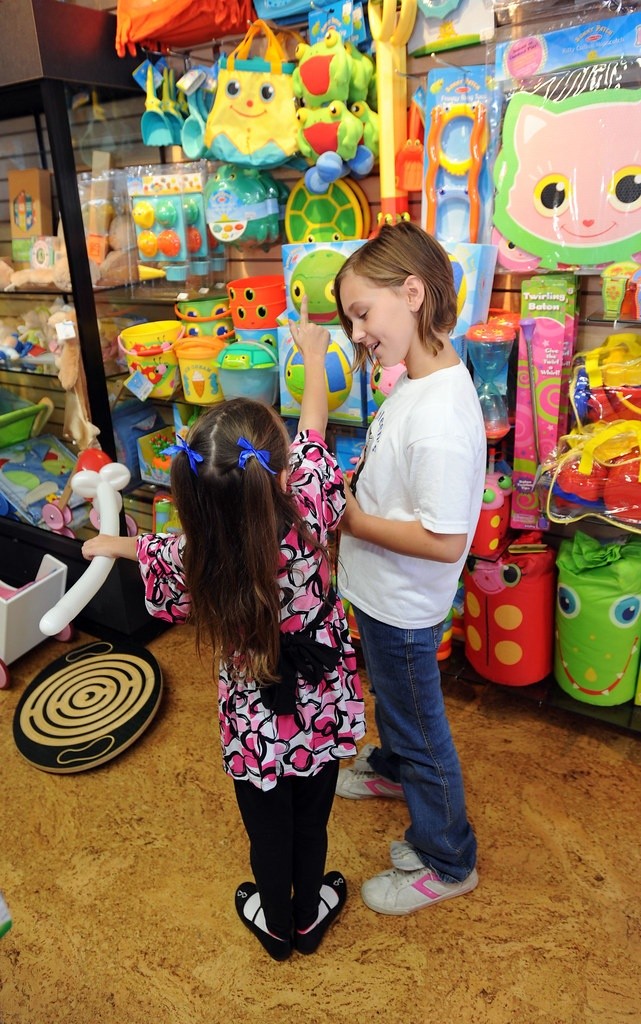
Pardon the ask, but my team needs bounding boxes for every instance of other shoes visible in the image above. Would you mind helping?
[235,882,293,962]
[293,871,347,955]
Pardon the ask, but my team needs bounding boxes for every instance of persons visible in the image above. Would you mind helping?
[334,221,488,915]
[80,294,347,962]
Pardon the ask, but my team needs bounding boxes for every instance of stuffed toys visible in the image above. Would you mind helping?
[1,213,138,443]
[291,28,379,194]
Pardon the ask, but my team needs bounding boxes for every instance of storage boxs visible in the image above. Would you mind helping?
[7,169,53,261]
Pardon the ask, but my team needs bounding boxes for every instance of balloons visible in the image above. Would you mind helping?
[39,448,132,636]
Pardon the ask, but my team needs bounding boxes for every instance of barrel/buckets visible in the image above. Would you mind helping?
[115,275,288,409]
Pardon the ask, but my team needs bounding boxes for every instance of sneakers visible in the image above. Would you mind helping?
[361,841,480,914]
[334,744,408,801]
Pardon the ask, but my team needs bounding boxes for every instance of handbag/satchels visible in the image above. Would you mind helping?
[203,19,313,171]
[541,333,641,535]
